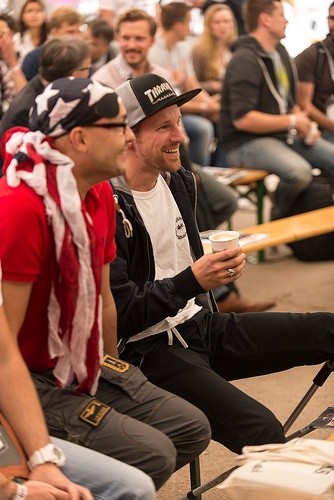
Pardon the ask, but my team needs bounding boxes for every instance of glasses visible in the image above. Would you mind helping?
[81,119,129,134]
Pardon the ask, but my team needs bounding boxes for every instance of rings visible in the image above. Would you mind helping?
[228,269,235,277]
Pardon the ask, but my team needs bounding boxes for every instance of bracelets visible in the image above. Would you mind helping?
[201,103,208,112]
[288,114,296,131]
[12,482,28,500]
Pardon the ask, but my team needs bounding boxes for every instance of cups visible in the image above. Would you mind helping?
[208,230,241,255]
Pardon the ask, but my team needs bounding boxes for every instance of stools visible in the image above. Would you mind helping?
[201,166,269,264]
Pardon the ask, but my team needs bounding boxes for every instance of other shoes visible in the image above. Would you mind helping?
[215,289,276,312]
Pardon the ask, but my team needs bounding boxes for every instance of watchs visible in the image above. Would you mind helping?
[26,443,66,472]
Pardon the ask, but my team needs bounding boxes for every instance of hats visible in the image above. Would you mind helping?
[115,73,203,129]
[27,76,120,138]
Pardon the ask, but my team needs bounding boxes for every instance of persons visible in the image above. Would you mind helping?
[115,76,334,457]
[0,76,212,500]
[0,0,334,262]
